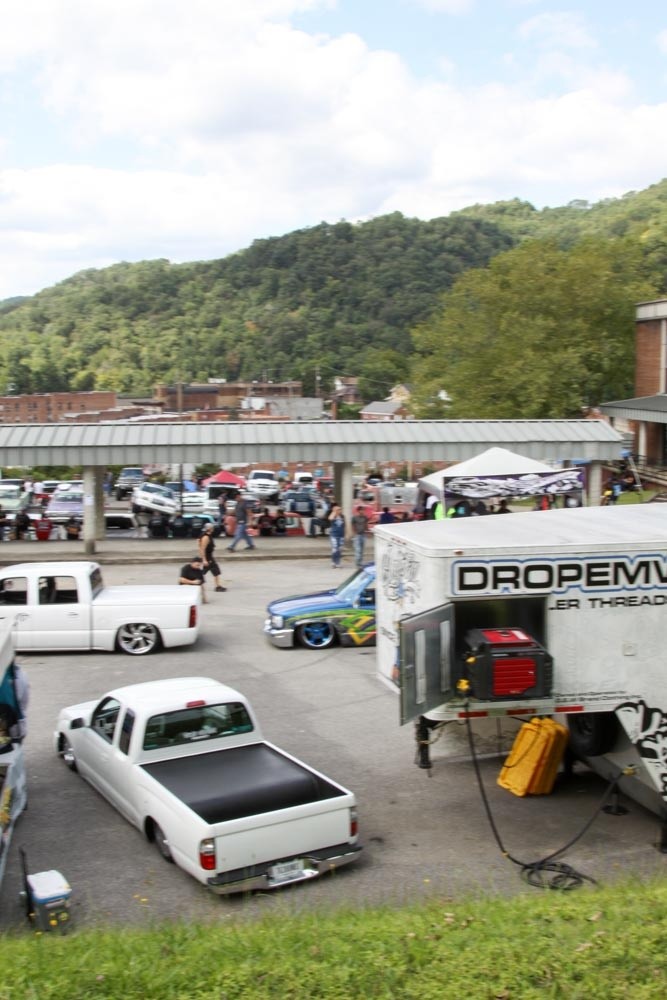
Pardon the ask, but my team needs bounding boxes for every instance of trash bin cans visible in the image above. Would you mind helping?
[29,870,72,931]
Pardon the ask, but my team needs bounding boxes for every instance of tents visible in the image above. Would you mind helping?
[415,445,588,519]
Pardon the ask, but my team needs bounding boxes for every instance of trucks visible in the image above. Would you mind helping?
[368,502,667,818]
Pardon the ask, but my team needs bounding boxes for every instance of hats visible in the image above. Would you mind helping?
[194,558,201,562]
[201,523,213,531]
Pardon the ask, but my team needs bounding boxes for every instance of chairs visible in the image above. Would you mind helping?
[39,586,49,604]
[160,707,205,735]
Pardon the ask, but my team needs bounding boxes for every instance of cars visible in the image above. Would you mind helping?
[263,562,376,650]
[113,467,419,536]
[0,479,85,523]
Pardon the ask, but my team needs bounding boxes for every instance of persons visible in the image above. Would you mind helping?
[602,461,639,505]
[380,507,395,524]
[210,489,231,537]
[351,506,369,568]
[179,557,209,604]
[328,506,345,569]
[24,478,47,504]
[222,491,256,552]
[169,512,191,538]
[307,502,338,538]
[270,508,287,538]
[190,515,203,538]
[199,523,226,592]
[1,504,81,543]
[148,509,168,539]
[257,508,274,537]
[447,498,512,516]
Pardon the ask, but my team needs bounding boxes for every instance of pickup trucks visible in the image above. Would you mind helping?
[0,561,201,656]
[55,677,363,895]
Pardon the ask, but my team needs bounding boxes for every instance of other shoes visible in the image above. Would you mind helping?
[227,548,233,552]
[216,587,226,592]
[246,545,254,550]
